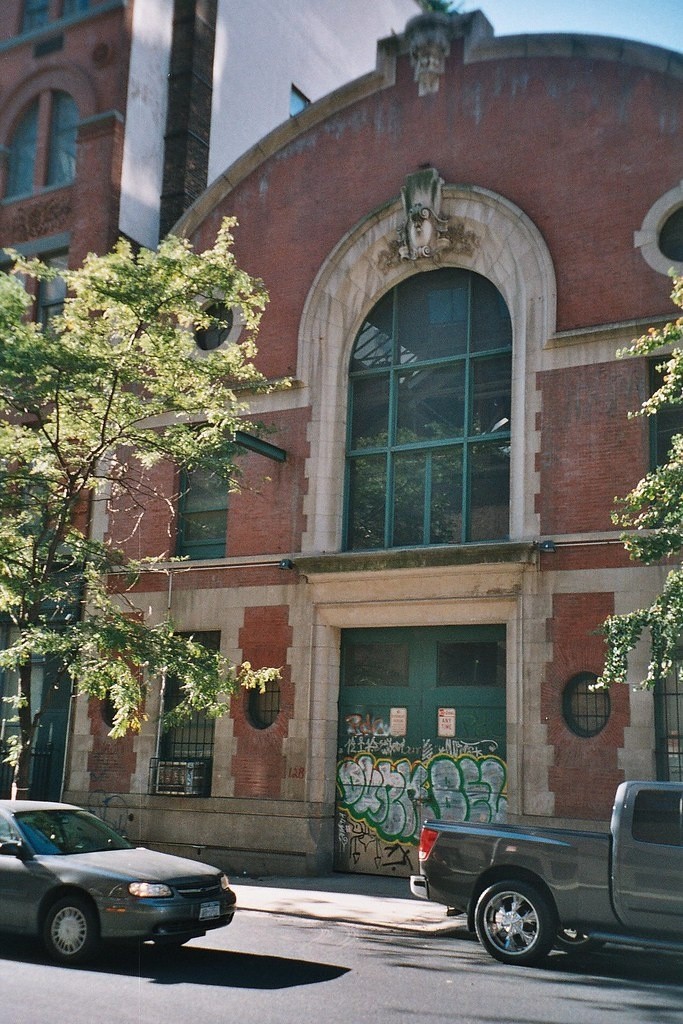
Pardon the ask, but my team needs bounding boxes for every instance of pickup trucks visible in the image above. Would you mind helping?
[409,781,683,966]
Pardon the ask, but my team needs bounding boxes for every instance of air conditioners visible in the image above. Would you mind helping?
[154,760,207,796]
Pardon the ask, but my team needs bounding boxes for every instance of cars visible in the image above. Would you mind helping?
[0,800,237,966]
